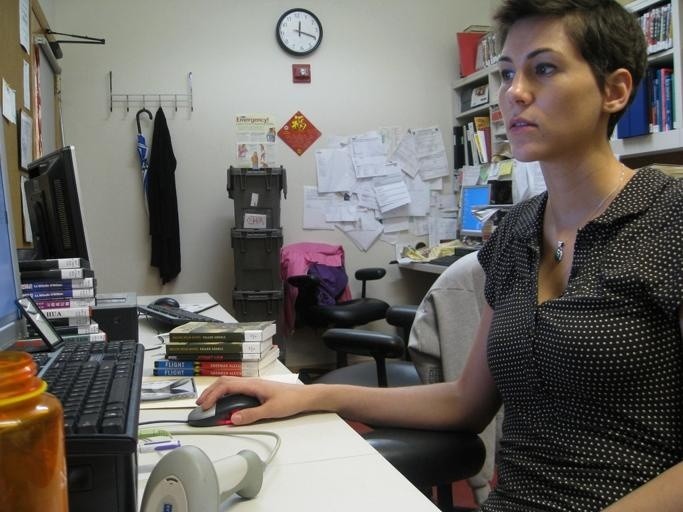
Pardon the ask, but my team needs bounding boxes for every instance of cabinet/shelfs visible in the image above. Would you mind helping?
[453,2,683,184]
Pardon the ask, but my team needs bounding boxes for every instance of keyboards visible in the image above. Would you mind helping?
[137,303,224,325]
[31,339,146,456]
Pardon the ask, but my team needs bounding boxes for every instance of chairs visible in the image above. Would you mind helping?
[310,301,440,431]
[281,240,392,382]
[358,430,487,512]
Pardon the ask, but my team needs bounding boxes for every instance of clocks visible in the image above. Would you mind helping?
[275,6,324,57]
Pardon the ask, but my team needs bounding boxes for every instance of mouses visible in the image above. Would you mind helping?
[154,297,179,307]
[187,394,261,427]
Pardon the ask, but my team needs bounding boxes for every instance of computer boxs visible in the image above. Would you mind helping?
[67,454,138,511]
[487,179,512,204]
[91,290,139,342]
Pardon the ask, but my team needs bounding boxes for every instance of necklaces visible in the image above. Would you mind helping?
[525,164,628,267]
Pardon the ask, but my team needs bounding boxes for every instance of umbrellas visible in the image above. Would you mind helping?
[132,107,152,218]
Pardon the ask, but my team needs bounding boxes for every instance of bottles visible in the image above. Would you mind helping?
[1,350,71,512]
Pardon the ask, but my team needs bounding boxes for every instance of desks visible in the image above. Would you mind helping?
[132,293,441,510]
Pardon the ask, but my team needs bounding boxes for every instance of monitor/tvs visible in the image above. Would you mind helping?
[23,144,93,270]
[459,184,492,237]
[0,112,27,353]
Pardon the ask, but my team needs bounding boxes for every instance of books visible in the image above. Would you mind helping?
[15,255,110,345]
[451,4,679,170]
[151,317,283,381]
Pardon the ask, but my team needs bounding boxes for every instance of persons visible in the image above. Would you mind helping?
[193,0,682,512]
[249,152,259,170]
[237,143,246,157]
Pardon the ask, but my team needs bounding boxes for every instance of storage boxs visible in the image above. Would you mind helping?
[226,166,286,362]
